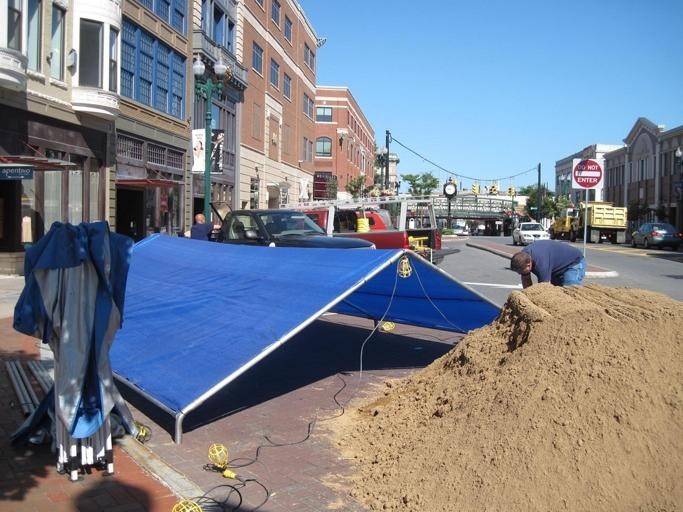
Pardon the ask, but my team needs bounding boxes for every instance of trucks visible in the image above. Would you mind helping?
[550,199,628,247]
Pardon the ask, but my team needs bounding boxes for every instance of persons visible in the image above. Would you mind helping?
[511,240,586,289]
[191,214,213,240]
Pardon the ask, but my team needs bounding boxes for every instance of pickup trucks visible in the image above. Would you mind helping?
[209,208,377,250]
[297,208,463,265]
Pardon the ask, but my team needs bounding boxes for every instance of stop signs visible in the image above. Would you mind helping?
[571,158,604,259]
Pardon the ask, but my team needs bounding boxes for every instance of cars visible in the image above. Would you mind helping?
[512,222,551,246]
[630,221,683,249]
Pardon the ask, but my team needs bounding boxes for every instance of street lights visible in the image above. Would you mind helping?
[193,46,227,225]
[372,146,388,191]
